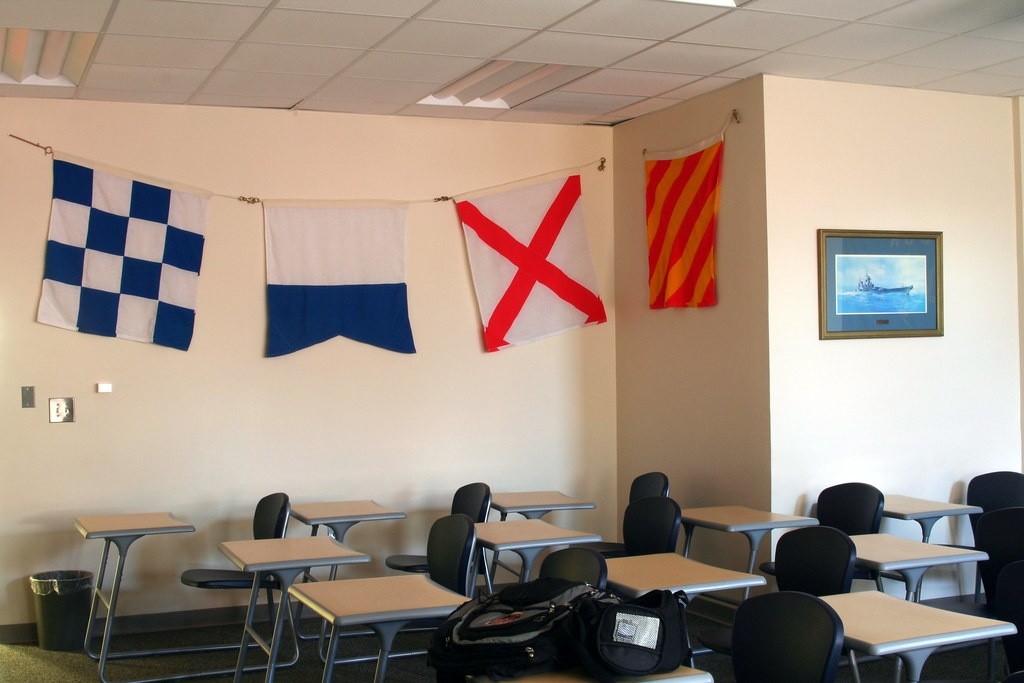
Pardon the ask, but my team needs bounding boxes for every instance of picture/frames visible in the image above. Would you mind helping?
[817,228,944,341]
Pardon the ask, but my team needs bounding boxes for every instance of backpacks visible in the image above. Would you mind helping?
[425,576,625,683]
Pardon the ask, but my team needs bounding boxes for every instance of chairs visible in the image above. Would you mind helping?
[99,492,301,683]
[385,483,494,627]
[540,472,682,589]
[697,485,883,683]
[968,472,1024,683]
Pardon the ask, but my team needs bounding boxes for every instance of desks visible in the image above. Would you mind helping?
[289,500,407,666]
[288,574,473,683]
[486,491,596,585]
[218,535,371,683]
[847,533,990,683]
[680,506,820,653]
[605,553,767,667]
[74,511,195,683]
[882,494,983,604]
[474,666,714,683]
[473,518,602,583]
[818,590,1018,683]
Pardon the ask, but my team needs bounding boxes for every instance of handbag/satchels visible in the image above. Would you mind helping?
[574,590,692,676]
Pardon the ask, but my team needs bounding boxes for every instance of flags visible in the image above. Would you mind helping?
[452,164,607,353]
[34,150,214,352]
[262,197,417,358]
[644,130,724,309]
